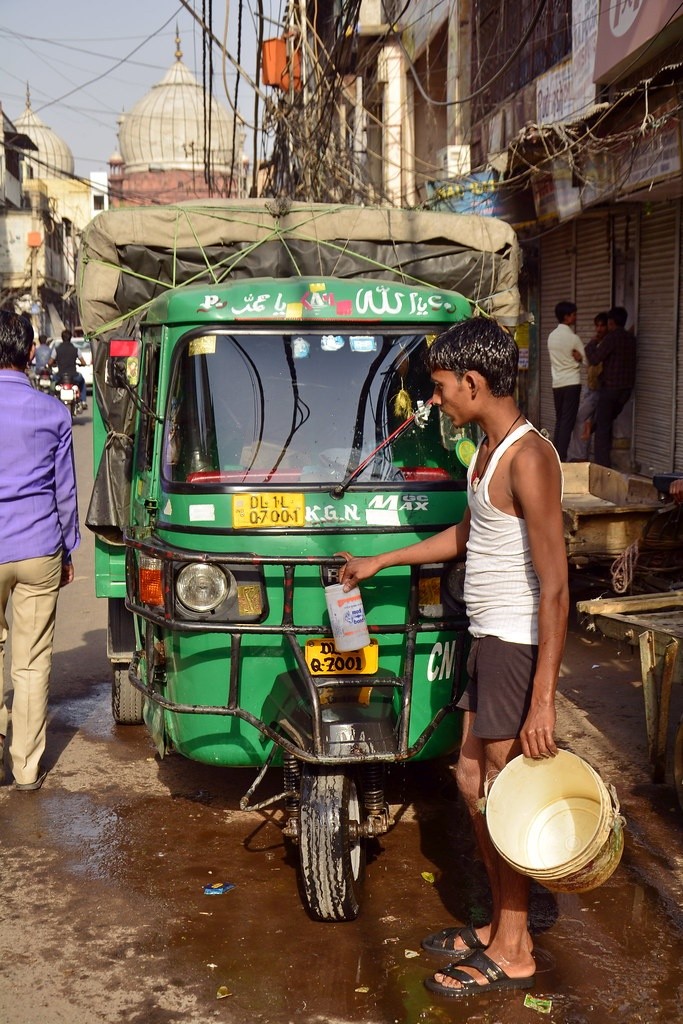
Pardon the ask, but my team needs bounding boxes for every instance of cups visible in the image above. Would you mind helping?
[324,584,371,652]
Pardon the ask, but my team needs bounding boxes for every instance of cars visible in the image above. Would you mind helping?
[49,338,94,387]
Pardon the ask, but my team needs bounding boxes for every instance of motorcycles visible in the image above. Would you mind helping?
[29,363,57,395]
[44,363,84,422]
[93,275,487,922]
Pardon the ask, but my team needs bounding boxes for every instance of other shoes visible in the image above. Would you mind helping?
[82,403,88,410]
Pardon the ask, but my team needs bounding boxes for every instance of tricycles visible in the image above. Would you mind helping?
[561,461,683,590]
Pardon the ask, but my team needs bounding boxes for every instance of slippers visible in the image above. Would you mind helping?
[16,764,47,791]
[422,923,490,954]
[0,743,7,785]
[425,949,536,997]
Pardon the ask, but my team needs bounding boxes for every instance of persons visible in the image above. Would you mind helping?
[567,307,636,468]
[547,301,590,463]
[26,335,52,374]
[332,313,569,998]
[49,330,86,409]
[0,310,81,790]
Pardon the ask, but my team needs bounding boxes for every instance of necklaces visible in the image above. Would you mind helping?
[471,412,522,493]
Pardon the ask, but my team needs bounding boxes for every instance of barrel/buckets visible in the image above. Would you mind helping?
[483,749,625,895]
